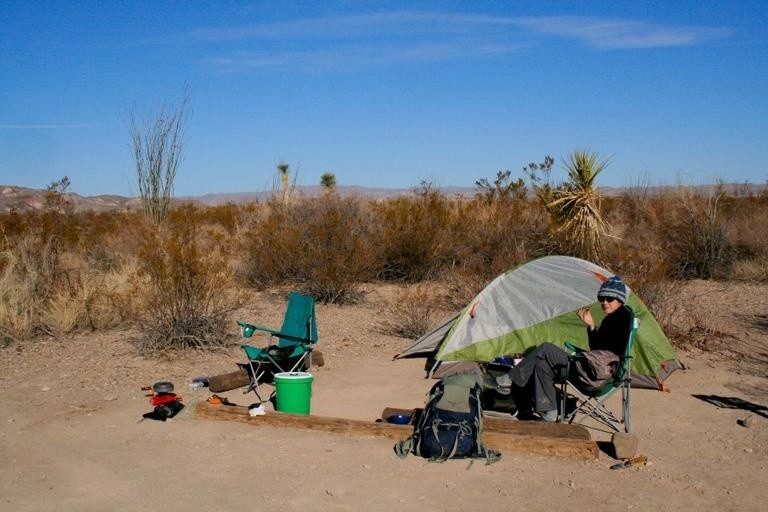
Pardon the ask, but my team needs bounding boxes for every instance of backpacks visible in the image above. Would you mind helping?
[393,372,504,466]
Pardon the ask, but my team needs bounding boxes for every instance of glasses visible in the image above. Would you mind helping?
[597,296,618,302]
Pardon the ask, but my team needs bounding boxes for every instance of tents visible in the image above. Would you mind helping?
[392,252,687,394]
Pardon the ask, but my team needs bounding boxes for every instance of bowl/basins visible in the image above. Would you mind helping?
[389,415,410,424]
[495,357,512,364]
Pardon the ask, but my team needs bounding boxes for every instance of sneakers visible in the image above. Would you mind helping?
[482,375,513,396]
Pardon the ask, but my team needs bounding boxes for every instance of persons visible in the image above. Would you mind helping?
[481,275,634,422]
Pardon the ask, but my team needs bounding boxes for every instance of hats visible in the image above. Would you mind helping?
[598,274,627,304]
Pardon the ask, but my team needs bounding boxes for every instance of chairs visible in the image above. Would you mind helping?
[559,315,640,433]
[236,291,318,405]
